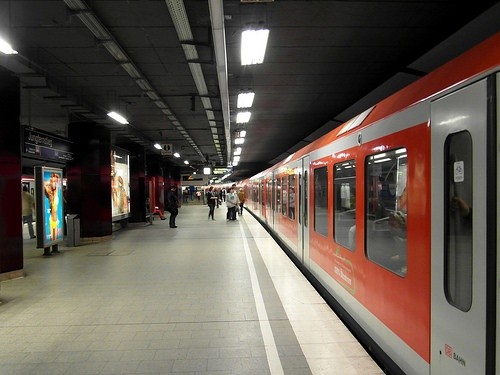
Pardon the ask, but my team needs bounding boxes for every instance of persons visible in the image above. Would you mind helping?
[206,186,225,220]
[368,195,407,272]
[112,177,128,215]
[22,185,36,239]
[165,184,179,228]
[225,184,245,220]
[451,198,472,232]
[45,174,60,241]
[282,187,295,220]
[183,188,201,202]
[153,207,166,220]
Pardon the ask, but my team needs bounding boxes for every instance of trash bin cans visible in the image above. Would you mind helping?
[67,214,80,247]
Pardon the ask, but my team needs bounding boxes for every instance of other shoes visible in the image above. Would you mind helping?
[170,226,177,228]
[31,236,36,239]
[232,219,236,220]
[161,218,166,220]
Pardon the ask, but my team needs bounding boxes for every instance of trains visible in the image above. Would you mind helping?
[203,32,499,375]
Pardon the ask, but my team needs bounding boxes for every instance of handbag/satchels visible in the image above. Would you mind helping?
[177,201,181,208]
